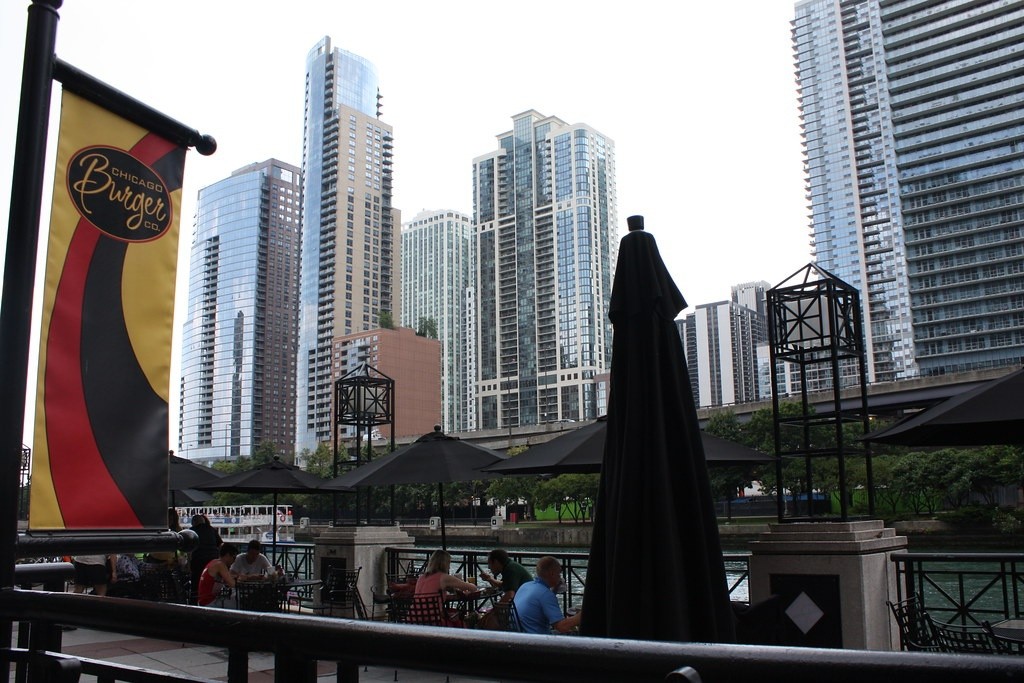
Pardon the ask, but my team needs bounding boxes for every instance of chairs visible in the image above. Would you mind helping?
[139,565,278,613]
[888,594,1024,656]
[370,573,452,628]
[485,587,524,633]
[300,566,362,615]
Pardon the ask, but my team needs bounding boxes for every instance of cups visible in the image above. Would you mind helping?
[467,577,475,584]
[266,566,275,575]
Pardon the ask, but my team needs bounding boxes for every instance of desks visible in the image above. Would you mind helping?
[225,578,323,588]
[982,619,1024,656]
[447,587,501,604]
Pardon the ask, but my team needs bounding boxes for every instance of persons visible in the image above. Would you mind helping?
[228,539,272,580]
[478,549,534,632]
[116,552,147,582]
[407,549,477,628]
[62,553,118,630]
[197,543,240,610]
[580,497,592,516]
[506,556,581,635]
[143,508,179,565]
[190,515,223,592]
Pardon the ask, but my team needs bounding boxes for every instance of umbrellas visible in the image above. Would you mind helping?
[188,454,358,566]
[852,367,1024,446]
[480,415,781,475]
[322,424,510,550]
[578,215,737,643]
[169,450,226,510]
